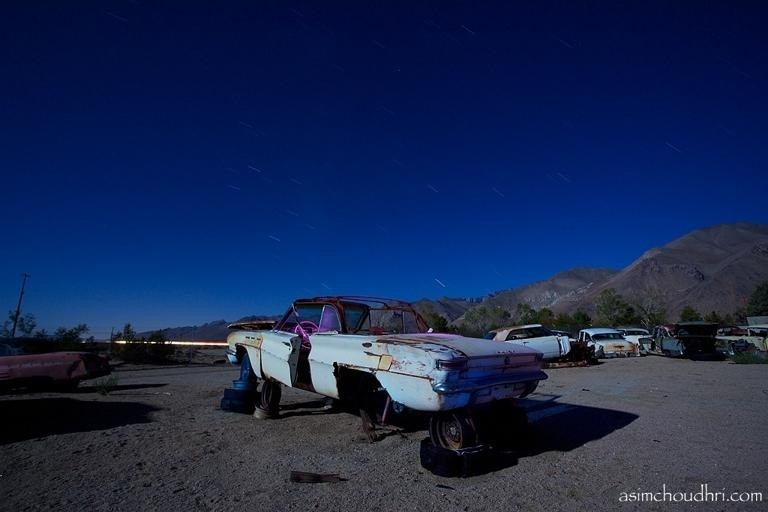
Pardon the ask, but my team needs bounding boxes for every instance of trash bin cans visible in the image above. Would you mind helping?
[735,344,745,356]
[586,349,594,363]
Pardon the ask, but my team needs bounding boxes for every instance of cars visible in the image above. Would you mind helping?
[476,321,600,369]
[222,293,549,456]
[574,325,642,360]
[616,327,656,353]
